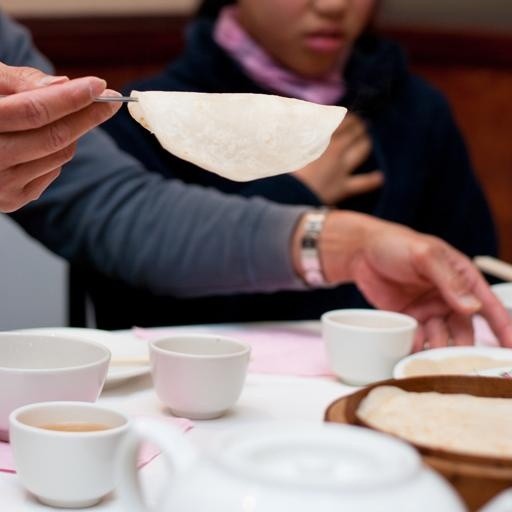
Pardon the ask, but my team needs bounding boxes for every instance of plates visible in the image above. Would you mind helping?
[16,327,151,389]
[393,344,512,383]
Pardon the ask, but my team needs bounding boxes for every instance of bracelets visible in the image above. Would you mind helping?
[300,203,339,288]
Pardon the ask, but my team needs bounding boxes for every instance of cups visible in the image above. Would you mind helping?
[0,333,134,508]
[145,333,249,421]
[320,306,418,389]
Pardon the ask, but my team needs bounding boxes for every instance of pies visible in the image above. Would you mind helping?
[127,89,349,182]
[357,384,512,458]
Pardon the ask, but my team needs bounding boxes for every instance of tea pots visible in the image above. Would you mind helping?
[116,420,511,512]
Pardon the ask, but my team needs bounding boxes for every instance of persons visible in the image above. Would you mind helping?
[1,0,511,355]
[89,0,507,331]
